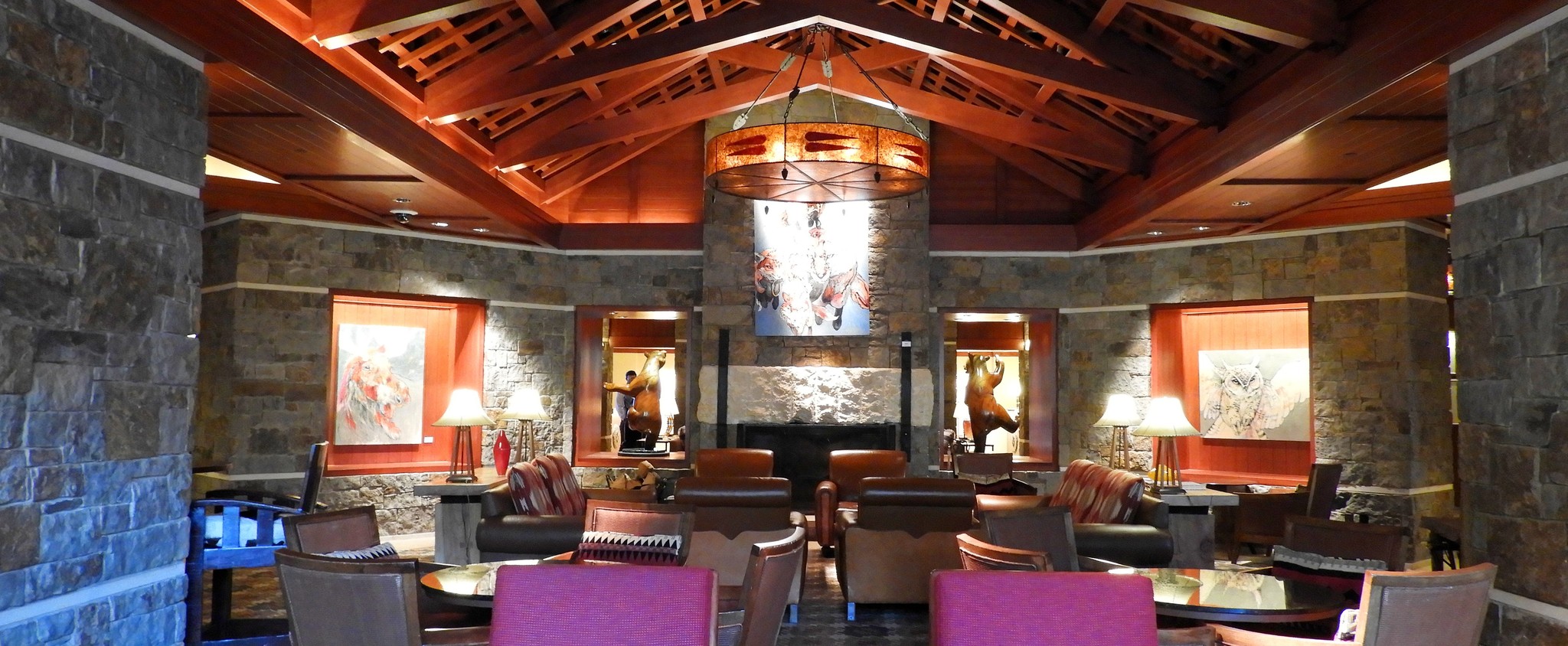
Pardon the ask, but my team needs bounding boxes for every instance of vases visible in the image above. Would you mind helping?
[493,429,511,476]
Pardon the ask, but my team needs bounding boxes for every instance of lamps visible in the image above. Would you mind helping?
[431,388,499,484]
[499,388,555,465]
[1131,397,1204,496]
[953,399,973,439]
[705,24,932,204]
[1094,394,1141,472]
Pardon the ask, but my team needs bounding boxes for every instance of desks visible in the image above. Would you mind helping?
[1130,471,1239,569]
[1107,566,1346,623]
[421,558,632,608]
[958,440,994,452]
[413,467,515,565]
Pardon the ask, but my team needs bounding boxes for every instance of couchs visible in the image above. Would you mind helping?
[674,475,809,621]
[833,476,982,624]
[975,460,1175,567]
[695,447,774,476]
[475,454,586,563]
[816,449,906,556]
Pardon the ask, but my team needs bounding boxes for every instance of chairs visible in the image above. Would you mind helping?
[929,462,1500,645]
[187,439,807,646]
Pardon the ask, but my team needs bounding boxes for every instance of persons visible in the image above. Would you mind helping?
[615,370,637,452]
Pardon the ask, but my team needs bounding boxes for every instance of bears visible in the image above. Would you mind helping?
[961,351,1021,453]
[601,349,667,451]
